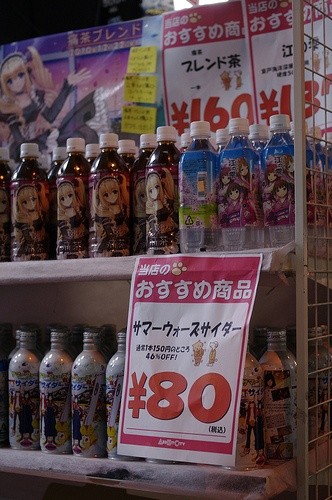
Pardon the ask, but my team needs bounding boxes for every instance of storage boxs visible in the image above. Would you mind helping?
[0,11,166,169]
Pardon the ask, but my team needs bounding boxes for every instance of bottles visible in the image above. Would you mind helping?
[0,115,331,263]
[1,321,332,472]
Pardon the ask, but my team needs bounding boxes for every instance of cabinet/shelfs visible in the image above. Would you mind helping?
[0,241,332,499]
